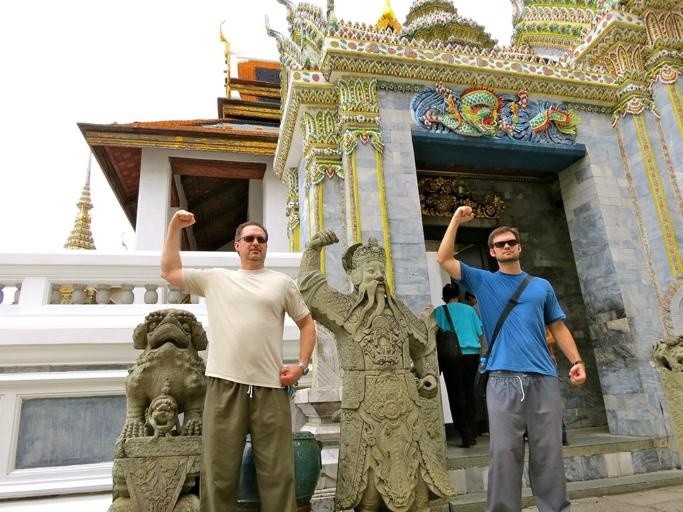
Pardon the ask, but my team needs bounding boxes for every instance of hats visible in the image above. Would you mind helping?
[441,283,460,299]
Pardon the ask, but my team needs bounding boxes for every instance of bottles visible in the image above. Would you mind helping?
[477,358,487,373]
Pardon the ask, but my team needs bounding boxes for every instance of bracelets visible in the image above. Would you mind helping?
[571,361,586,366]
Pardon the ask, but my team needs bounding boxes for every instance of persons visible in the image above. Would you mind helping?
[433,201,590,512]
[296,227,459,512]
[429,280,490,449]
[462,289,483,323]
[156,205,320,511]
[544,323,572,447]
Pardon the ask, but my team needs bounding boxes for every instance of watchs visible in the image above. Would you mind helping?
[298,363,308,376]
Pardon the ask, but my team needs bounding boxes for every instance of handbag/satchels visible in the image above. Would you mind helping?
[436,304,464,373]
[470,367,491,427]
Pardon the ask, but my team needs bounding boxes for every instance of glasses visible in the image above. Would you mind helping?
[491,239,519,249]
[237,235,268,245]
